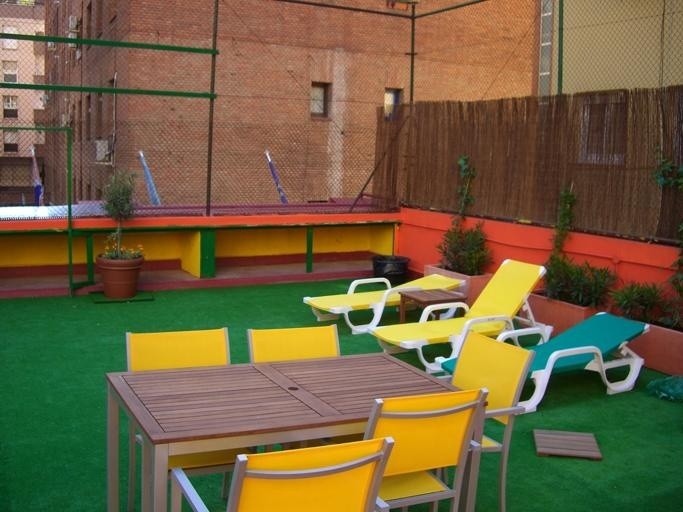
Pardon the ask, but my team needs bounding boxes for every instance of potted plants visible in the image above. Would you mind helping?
[606,147,683,377]
[424,155,493,317]
[98,171,145,298]
[519,188,618,337]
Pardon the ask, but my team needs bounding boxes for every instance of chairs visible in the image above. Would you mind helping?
[303,274,468,335]
[127,326,231,507]
[362,386,490,510]
[432,310,649,415]
[368,258,554,376]
[171,437,396,511]
[400,327,534,512]
[246,325,341,452]
